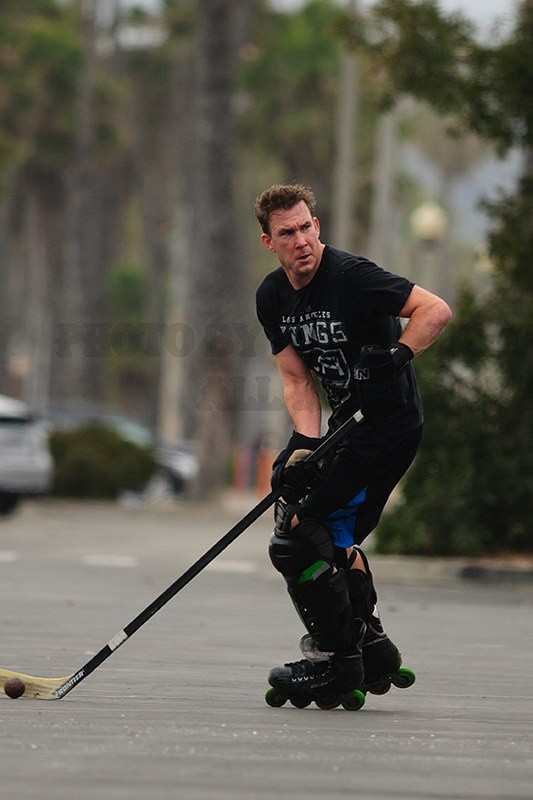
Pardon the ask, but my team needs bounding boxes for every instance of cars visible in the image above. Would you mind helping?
[96,417,199,506]
[0,396,53,516]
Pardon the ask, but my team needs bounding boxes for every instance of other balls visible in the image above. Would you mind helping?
[4,678,25,699]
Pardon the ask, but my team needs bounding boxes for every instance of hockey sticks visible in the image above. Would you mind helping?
[0,402,375,701]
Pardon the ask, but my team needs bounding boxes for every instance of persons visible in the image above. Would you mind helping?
[251,182,453,713]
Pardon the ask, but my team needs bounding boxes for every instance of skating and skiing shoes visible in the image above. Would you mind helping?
[265,619,367,711]
[315,615,415,710]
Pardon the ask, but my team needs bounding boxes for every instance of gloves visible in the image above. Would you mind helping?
[353,341,415,398]
[268,428,328,503]
